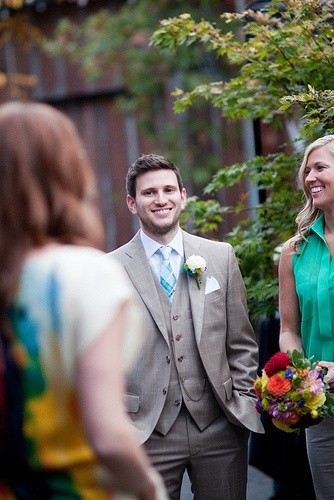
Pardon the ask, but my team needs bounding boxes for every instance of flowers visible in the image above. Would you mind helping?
[183,254,207,291]
[253,348,334,432]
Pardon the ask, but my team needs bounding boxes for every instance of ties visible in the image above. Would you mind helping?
[159,246,177,302]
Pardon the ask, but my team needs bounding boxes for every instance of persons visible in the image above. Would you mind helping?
[277,134,334,500]
[0,99,173,500]
[101,152,266,500]
